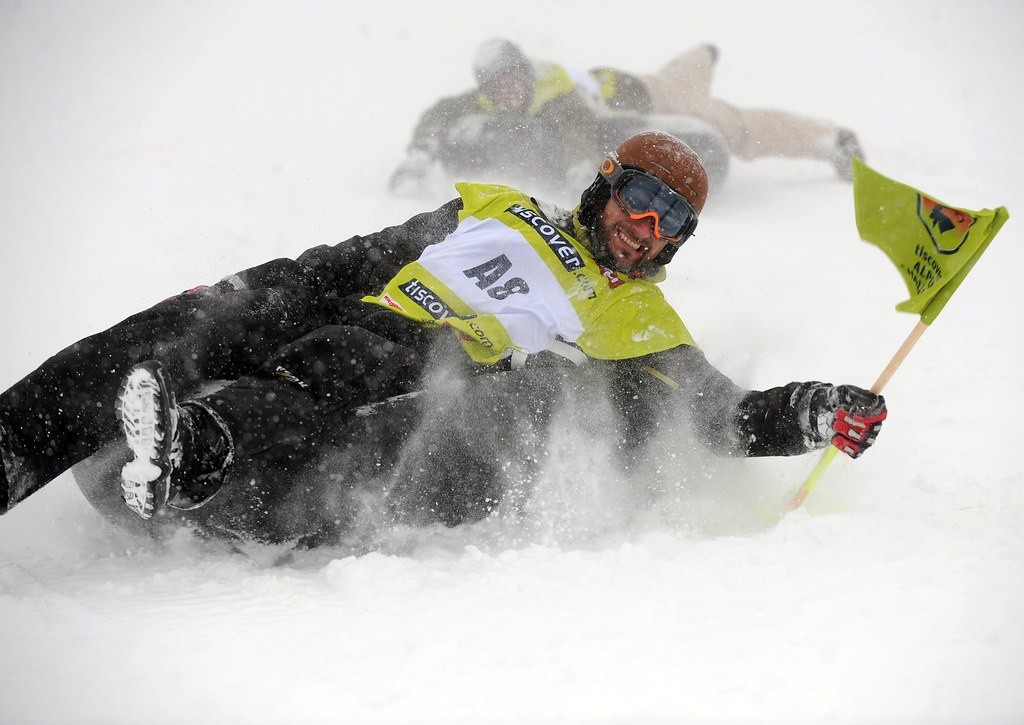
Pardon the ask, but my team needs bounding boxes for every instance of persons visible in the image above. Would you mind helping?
[0,131,887,556]
[388,40,864,202]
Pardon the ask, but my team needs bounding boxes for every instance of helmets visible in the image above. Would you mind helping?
[578,131,709,266]
[472,39,527,88]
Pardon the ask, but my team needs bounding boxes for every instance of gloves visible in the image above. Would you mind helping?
[386,148,432,194]
[182,281,237,296]
[794,383,887,459]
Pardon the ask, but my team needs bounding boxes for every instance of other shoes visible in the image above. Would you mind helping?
[833,128,865,178]
[702,38,720,67]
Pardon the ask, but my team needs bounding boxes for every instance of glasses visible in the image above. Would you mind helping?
[611,169,698,248]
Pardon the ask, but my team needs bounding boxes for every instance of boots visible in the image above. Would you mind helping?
[115,361,226,521]
